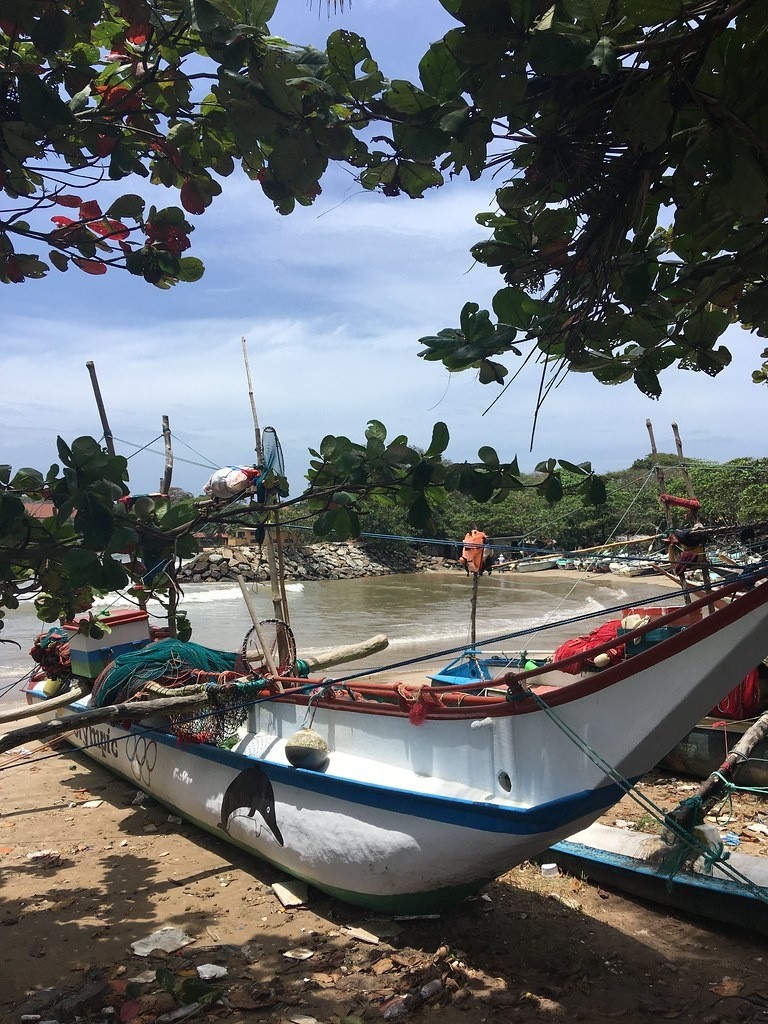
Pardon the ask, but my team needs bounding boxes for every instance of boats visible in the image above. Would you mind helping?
[19,339,768,920]
[554,548,762,583]
[516,554,563,575]
[659,715,768,790]
